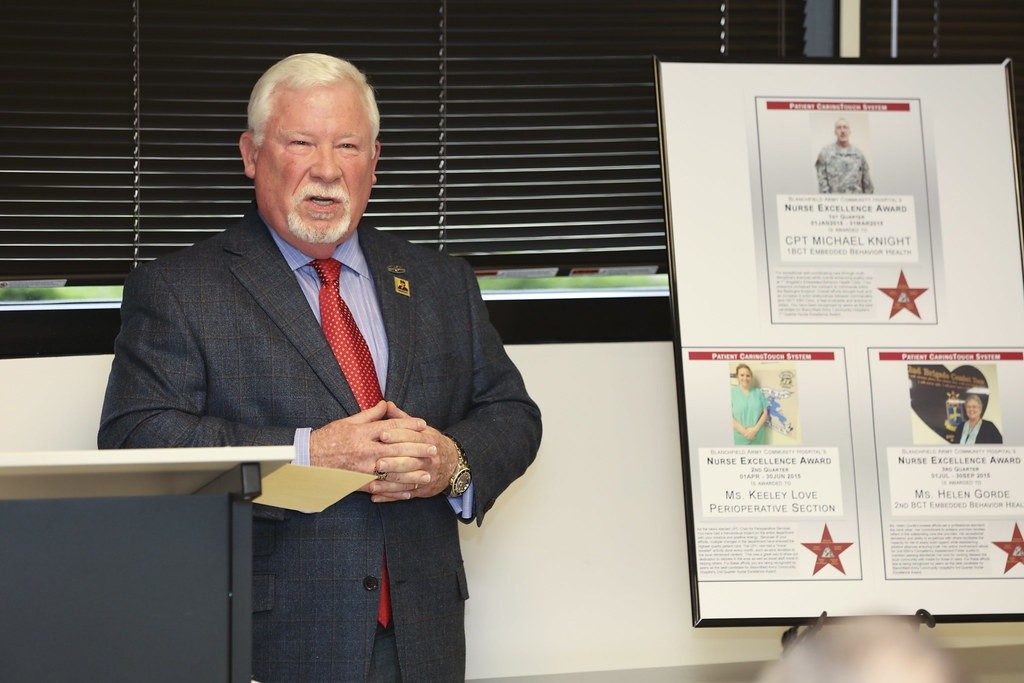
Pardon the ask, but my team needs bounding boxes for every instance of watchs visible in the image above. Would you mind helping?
[444,434,472,499]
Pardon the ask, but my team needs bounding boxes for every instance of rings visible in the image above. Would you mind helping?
[414,483,418,489]
[373,467,387,480]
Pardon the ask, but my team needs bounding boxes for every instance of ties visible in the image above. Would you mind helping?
[308,259,391,627]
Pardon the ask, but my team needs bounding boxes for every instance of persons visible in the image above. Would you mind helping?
[97,51,543,683]
[732,364,768,448]
[952,396,1004,443]
[815,118,875,194]
